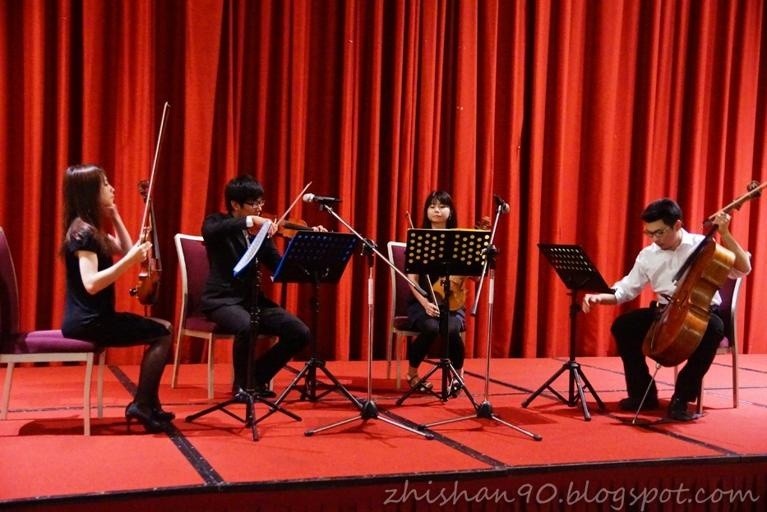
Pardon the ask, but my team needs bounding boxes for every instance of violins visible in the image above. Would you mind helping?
[432,216,491,312]
[129,181,164,303]
[246,213,335,239]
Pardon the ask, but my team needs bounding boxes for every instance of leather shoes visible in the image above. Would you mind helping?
[619,397,660,410]
[668,393,687,418]
[231,382,276,401]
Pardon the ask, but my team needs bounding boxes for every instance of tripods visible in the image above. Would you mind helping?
[304,240,437,444]
[182,277,302,442]
[521,293,626,422]
[268,282,367,414]
[395,275,491,415]
[415,250,544,442]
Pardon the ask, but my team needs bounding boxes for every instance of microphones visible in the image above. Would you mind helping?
[492,193,511,221]
[298,192,344,210]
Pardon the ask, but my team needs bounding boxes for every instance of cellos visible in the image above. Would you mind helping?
[642,181,760,367]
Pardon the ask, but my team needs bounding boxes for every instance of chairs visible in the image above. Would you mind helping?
[674,268,742,415]
[170,233,277,401]
[387,242,419,392]
[0,228,105,437]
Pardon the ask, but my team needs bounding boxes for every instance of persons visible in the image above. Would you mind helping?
[582,198,751,421]
[61,164,175,434]
[405,190,466,397]
[201,173,311,402]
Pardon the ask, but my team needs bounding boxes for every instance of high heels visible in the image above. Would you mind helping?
[125,402,175,434]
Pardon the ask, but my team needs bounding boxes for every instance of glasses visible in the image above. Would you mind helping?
[642,222,675,239]
[244,199,265,209]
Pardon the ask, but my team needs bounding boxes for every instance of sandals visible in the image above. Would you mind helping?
[406,372,433,393]
[451,376,464,398]
